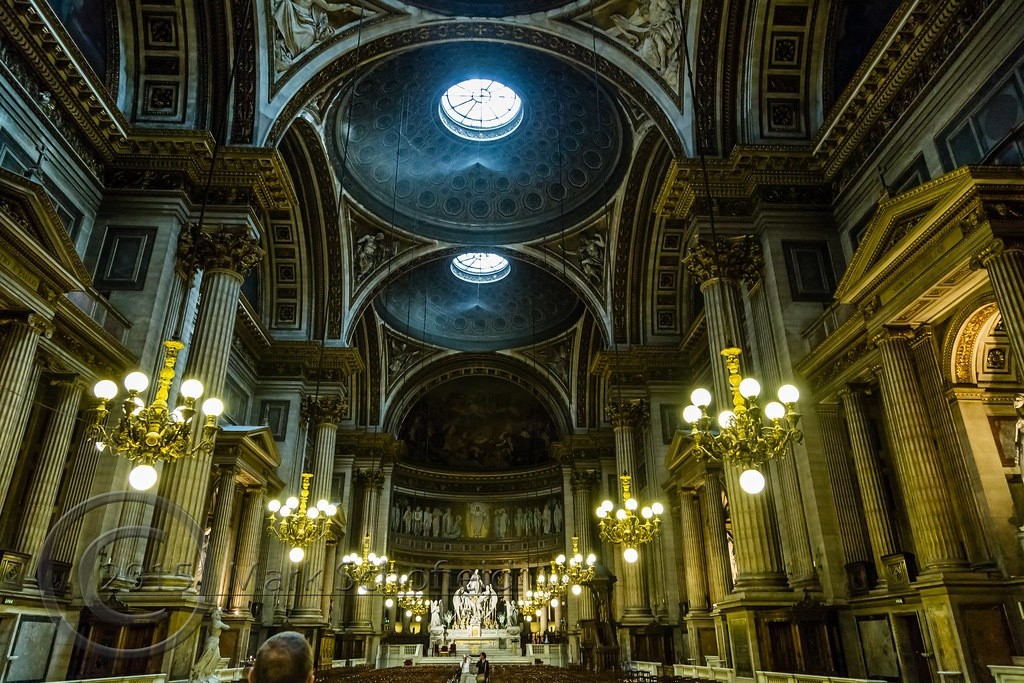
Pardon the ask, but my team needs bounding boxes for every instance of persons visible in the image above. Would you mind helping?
[460,652,471,683]
[190,610,230,679]
[247,631,314,683]
[391,501,563,537]
[476,650,489,683]
[428,570,519,628]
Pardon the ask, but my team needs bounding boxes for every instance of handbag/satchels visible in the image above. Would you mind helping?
[475,674,486,683]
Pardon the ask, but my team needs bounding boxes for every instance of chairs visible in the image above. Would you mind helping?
[315,663,721,683]
[440,646,449,657]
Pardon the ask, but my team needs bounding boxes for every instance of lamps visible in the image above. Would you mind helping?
[556,0,598,593]
[264,0,366,561]
[398,1,441,619]
[344,0,416,594]
[376,0,431,608]
[678,0,805,494]
[588,0,665,563]
[89,0,251,491]
[520,97,567,621]
